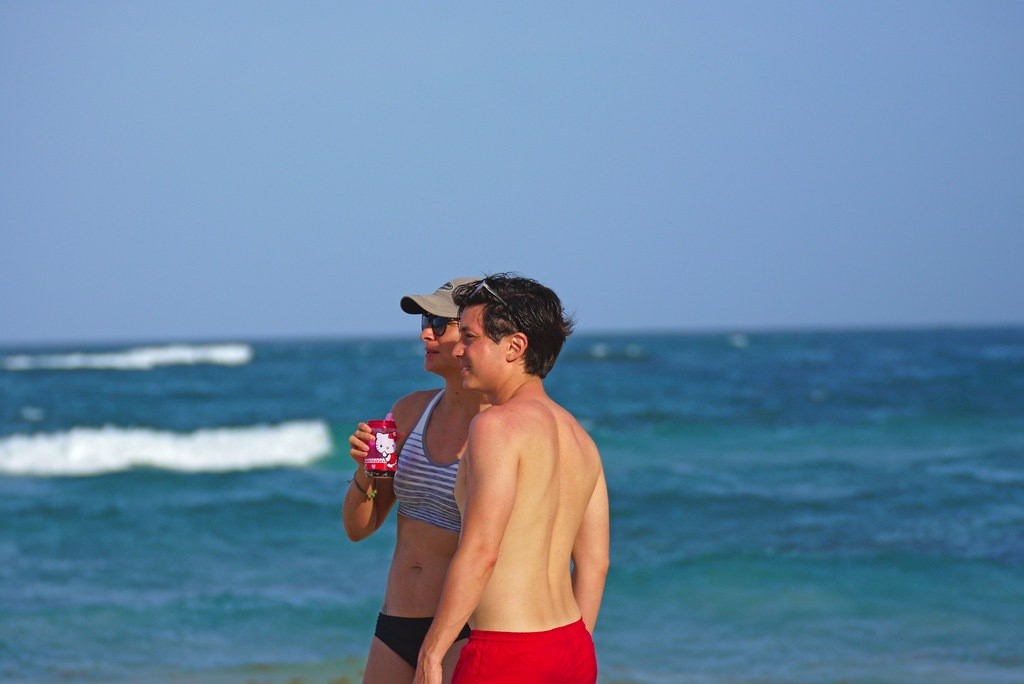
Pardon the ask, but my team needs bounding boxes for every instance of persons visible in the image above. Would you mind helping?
[343,277,492,684]
[414,276,609,684]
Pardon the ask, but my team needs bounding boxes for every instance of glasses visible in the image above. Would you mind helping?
[465,279,508,308]
[421,313,458,337]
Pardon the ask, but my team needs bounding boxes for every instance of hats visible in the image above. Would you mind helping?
[400,277,485,318]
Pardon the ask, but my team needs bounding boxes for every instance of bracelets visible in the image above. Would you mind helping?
[347,471,378,499]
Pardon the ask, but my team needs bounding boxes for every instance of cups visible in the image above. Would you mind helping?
[365,420,397,478]
